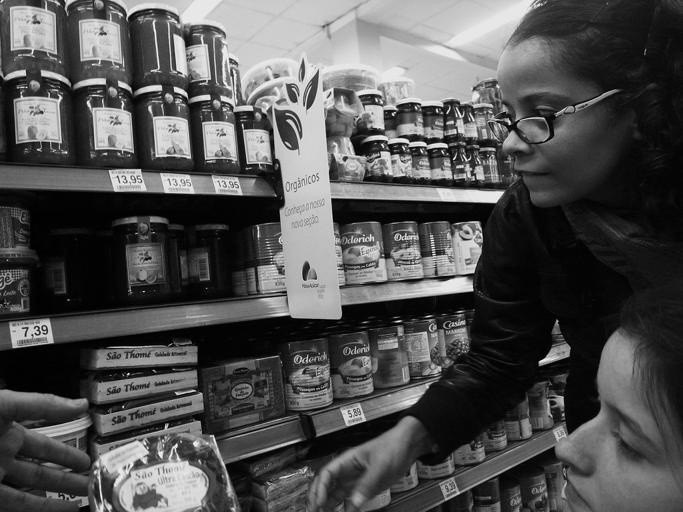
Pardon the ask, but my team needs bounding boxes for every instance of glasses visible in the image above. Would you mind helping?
[486,88,626,145]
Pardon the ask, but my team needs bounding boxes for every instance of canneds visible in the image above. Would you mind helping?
[278,310,475,411]
[354,371,566,512]
[330,219,485,285]
[347,82,516,189]
[0,1,278,175]
[1,203,288,317]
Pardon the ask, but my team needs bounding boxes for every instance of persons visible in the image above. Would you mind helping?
[555,286,682,511]
[1,390,94,511]
[307,1,683,512]
